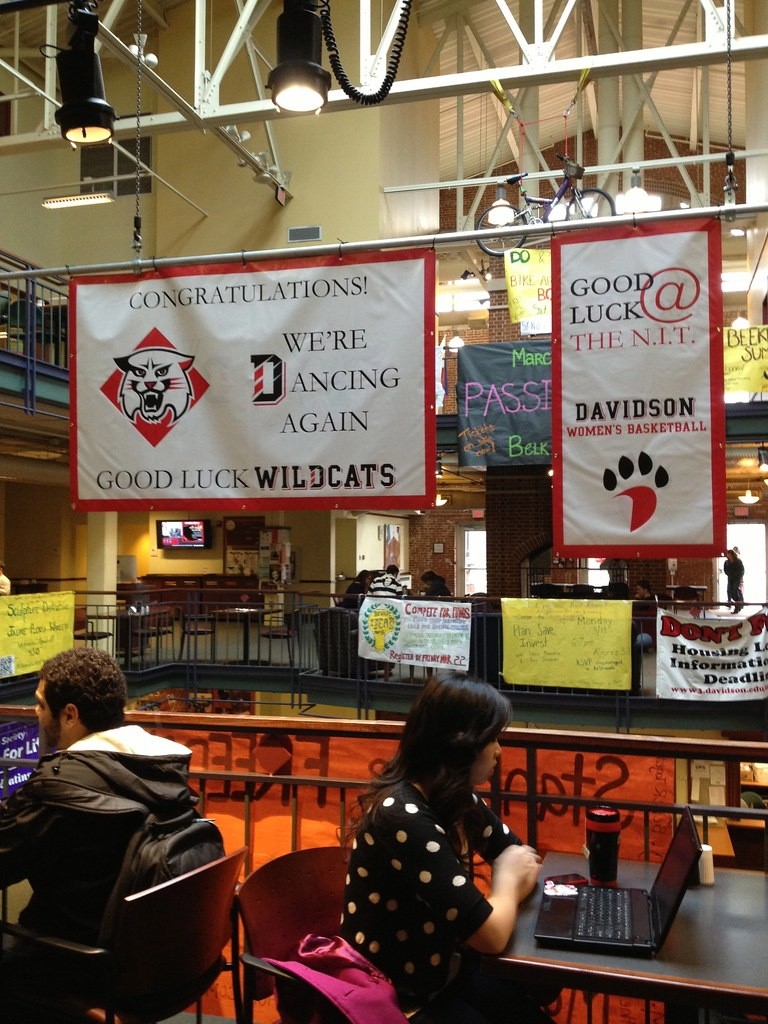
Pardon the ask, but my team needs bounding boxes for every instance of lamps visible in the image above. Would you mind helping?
[436,453,445,479]
[758,441,768,472]
[731,304,752,331]
[436,491,448,506]
[447,280,465,352]
[737,470,760,504]
[264,0,333,114]
[487,175,515,225]
[613,170,661,215]
[39,1,117,143]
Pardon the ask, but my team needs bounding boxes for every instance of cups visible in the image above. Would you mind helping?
[585,806,621,888]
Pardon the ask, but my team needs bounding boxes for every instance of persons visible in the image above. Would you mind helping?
[340,569,373,615]
[270,569,280,584]
[0,646,193,1024]
[724,546,746,614]
[419,571,451,597]
[0,561,11,596]
[635,581,655,610]
[332,670,563,1024]
[366,565,402,596]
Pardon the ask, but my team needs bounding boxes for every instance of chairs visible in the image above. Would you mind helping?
[227,848,412,1024]
[264,605,302,666]
[132,605,176,665]
[674,586,702,618]
[74,622,116,659]
[178,608,213,664]
[0,842,255,1024]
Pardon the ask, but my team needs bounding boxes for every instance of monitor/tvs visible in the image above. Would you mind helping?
[156,519,213,550]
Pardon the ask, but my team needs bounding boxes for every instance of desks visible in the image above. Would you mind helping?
[500,849,767,1024]
[211,607,265,670]
[87,610,170,674]
[666,585,707,617]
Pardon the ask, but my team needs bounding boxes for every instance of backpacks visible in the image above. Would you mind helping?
[13,778,225,947]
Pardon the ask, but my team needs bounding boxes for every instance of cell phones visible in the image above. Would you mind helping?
[544,873,589,885]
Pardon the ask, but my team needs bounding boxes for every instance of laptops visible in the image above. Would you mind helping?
[534,805,702,956]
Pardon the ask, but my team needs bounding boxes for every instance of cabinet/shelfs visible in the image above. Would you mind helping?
[203,579,263,621]
[143,581,199,616]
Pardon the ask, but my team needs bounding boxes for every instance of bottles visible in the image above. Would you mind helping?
[698,844,714,885]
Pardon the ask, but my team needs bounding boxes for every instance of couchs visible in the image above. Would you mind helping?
[317,606,396,678]
